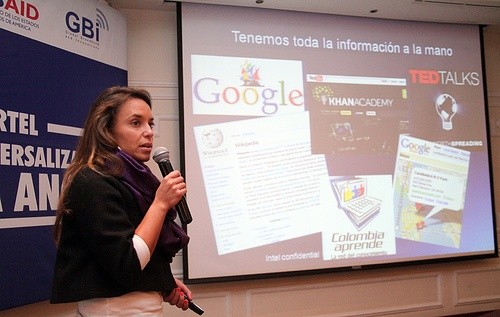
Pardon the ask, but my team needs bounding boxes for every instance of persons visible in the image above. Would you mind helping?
[50,85,193,312]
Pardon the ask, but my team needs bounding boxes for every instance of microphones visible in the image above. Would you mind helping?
[152,147,192,225]
